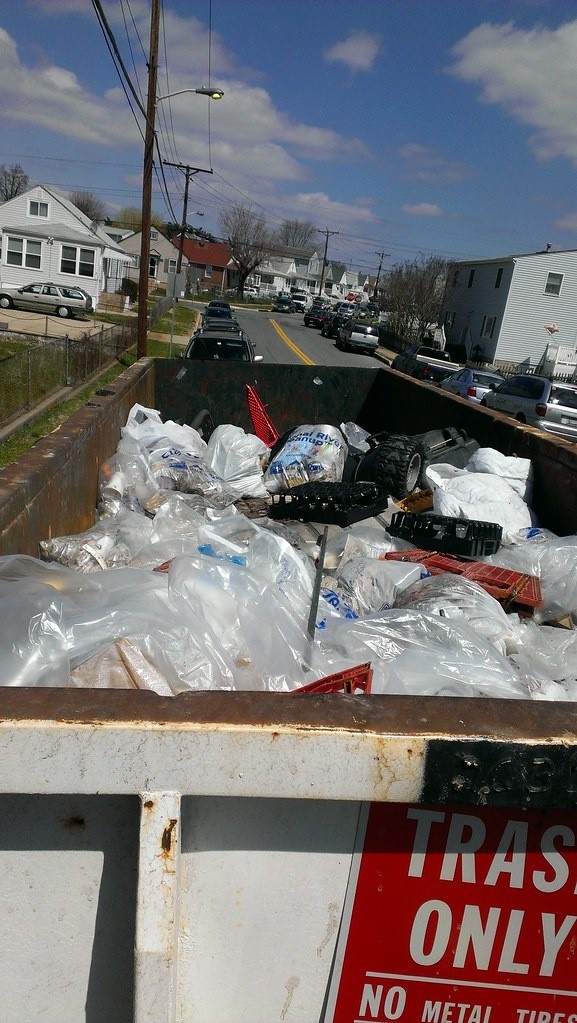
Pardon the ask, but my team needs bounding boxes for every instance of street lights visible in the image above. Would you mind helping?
[136,87,225,362]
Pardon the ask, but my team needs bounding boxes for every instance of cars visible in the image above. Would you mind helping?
[437,367,507,405]
[180,285,263,363]
[271,282,381,338]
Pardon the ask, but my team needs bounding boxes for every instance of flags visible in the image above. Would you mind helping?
[546,323,559,335]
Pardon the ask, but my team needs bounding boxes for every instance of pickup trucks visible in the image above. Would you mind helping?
[389,345,468,385]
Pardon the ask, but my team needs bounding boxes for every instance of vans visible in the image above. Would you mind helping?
[480,373,577,445]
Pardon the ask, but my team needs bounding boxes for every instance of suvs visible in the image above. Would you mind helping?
[335,320,381,357]
[0,282,94,319]
